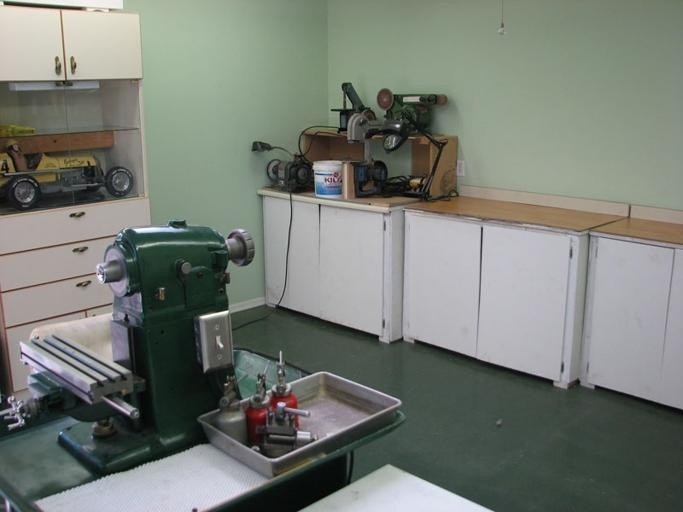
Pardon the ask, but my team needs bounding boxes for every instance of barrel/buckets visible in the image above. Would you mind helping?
[313,160,345,198]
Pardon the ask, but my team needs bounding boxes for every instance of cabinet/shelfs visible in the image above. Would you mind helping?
[403,202,621,389]
[263,198,404,345]
[0,4,144,81]
[1,196,151,395]
[578,218,682,410]
